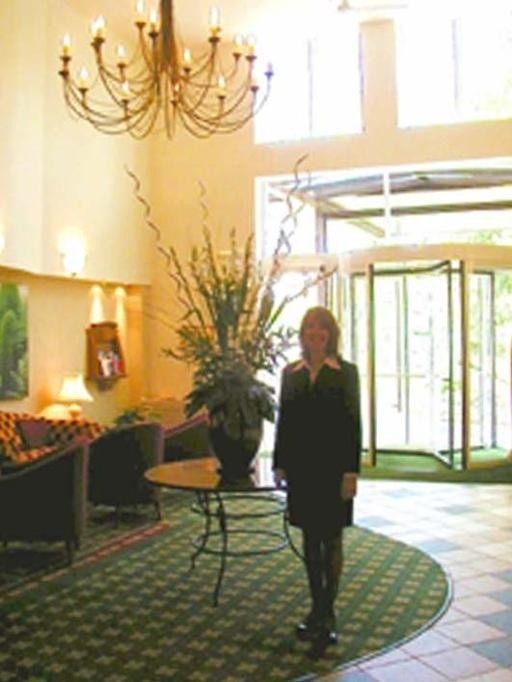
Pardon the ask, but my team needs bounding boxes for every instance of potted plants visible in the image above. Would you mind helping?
[114,153,342,477]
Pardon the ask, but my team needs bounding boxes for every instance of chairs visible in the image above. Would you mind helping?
[2,413,209,572]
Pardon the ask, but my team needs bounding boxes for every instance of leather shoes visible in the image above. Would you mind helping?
[296,612,338,644]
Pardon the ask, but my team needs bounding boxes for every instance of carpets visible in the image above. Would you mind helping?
[2,486,463,682]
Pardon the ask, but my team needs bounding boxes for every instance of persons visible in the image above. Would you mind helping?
[272,305,362,645]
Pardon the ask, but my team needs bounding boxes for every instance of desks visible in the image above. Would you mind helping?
[142,457,310,609]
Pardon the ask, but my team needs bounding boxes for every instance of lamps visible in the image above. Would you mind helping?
[59,374,94,419]
[58,0,283,143]
